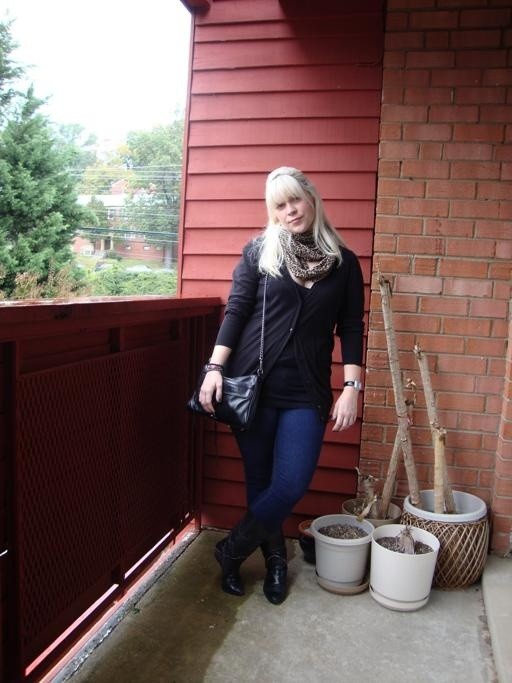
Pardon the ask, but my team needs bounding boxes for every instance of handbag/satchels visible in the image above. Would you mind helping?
[183,363,262,432]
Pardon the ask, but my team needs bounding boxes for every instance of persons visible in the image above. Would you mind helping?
[182,165,364,606]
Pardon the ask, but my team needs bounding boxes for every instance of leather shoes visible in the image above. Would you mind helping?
[262,556,287,605]
[213,542,246,597]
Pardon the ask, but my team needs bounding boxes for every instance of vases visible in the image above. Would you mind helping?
[298,486,489,610]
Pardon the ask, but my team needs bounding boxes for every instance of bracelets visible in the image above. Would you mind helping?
[204,362,224,372]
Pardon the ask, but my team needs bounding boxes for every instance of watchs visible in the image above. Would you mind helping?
[343,379,361,390]
